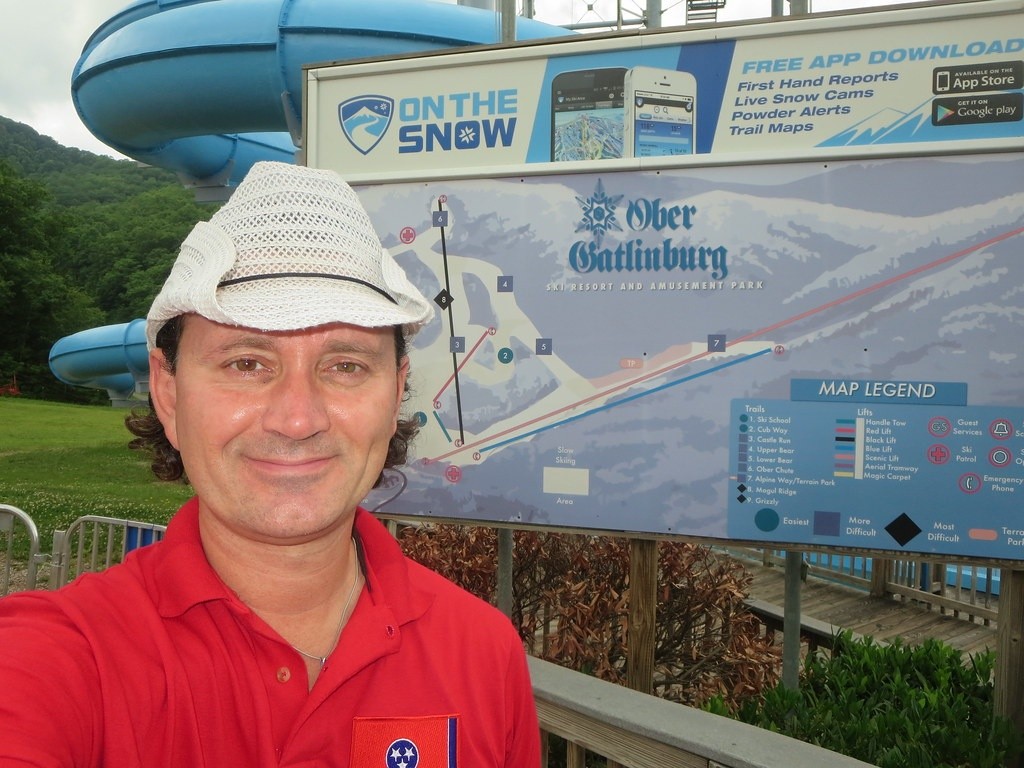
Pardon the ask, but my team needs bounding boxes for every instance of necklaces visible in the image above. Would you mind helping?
[211,530,358,666]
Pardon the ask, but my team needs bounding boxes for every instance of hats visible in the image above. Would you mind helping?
[147,161,436,351]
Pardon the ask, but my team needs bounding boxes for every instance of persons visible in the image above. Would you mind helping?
[0,161,541,768]
